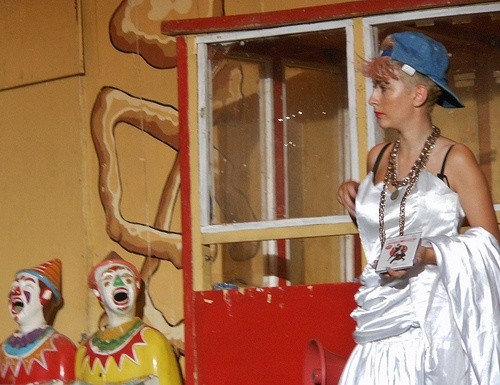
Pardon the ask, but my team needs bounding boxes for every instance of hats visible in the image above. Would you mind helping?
[378,32,465,109]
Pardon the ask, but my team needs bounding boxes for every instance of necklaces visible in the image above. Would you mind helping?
[378,125,440,253]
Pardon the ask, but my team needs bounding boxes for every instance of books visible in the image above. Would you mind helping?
[375,231,423,276]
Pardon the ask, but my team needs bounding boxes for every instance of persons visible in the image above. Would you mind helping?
[1,258,78,385]
[335,33,500,383]
[72,250,183,385]
